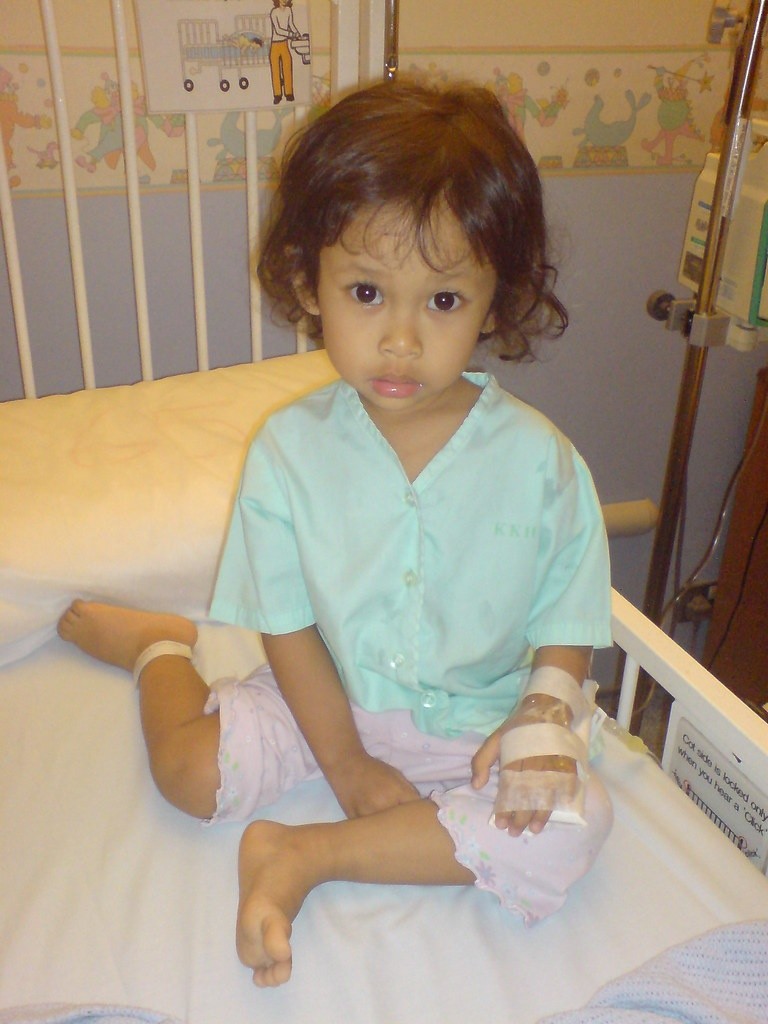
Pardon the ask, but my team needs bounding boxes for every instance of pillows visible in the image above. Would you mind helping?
[0,351,346,657]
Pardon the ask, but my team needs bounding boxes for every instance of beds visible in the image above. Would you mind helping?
[0,578,767,1024]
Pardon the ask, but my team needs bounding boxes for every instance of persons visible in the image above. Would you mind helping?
[59,71,614,989]
